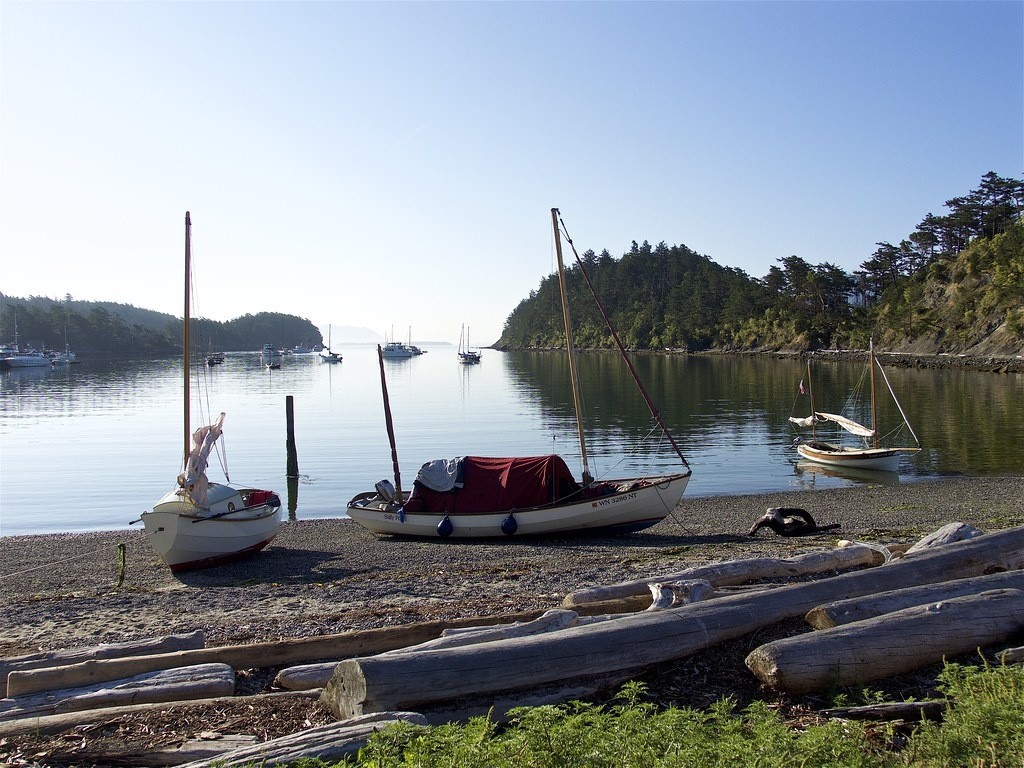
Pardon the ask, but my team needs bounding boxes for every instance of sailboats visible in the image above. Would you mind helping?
[343,207,693,540]
[788,332,922,473]
[456,322,481,364]
[318,323,343,363]
[132,211,282,567]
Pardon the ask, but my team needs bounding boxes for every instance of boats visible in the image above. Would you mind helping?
[265,361,280,369]
[0,306,81,367]
[260,341,325,356]
[376,322,424,358]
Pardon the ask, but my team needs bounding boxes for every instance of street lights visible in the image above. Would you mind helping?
[204,330,225,365]
